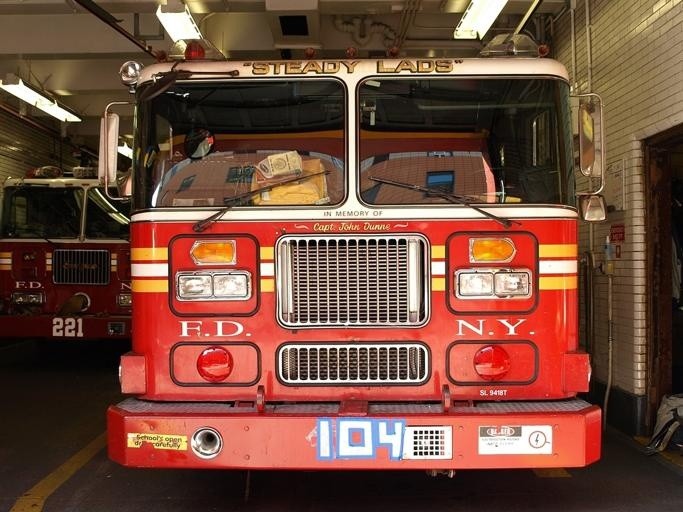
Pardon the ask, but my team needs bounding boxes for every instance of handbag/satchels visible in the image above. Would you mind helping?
[250,157,329,206]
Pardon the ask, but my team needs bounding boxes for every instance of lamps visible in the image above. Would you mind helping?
[156,0,204,44]
[0,55,83,123]
[453,0,509,40]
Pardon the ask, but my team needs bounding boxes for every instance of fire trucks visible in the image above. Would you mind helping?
[96,34,605,470]
[0,166,130,349]
